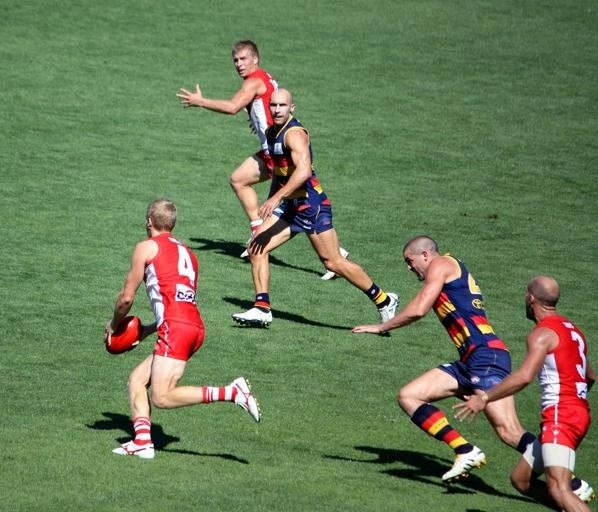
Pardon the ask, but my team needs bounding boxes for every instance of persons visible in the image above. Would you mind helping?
[175,41,349,283]
[101,197,262,459]
[229,89,400,328]
[350,237,595,511]
[452,274,596,512]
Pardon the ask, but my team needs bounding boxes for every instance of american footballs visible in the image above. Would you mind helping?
[105,315,141,355]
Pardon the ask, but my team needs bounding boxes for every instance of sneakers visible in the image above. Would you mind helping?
[111,440,154,459]
[378,293,400,335]
[321,246,350,281]
[569,475,595,504]
[230,377,261,424]
[442,446,487,482]
[240,232,260,258]
[232,308,272,328]
